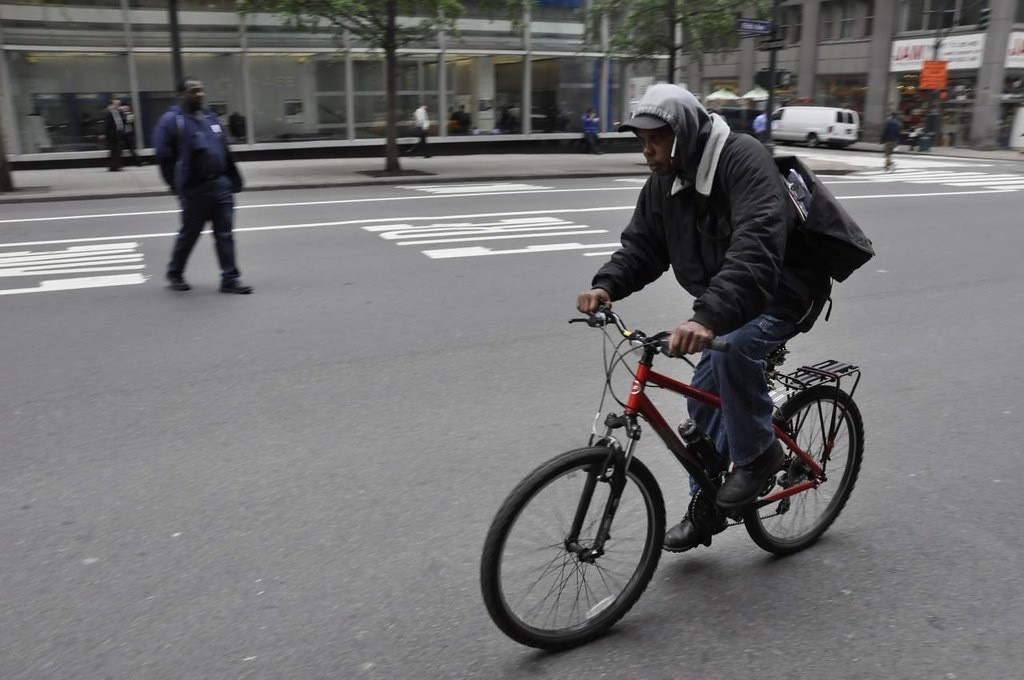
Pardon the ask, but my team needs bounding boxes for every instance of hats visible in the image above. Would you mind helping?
[617,112,668,133]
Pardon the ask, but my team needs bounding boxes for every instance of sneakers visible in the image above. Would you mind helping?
[218,277,254,294]
[166,267,190,290]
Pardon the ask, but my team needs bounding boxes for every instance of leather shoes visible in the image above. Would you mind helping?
[715,437,787,510]
[662,503,729,553]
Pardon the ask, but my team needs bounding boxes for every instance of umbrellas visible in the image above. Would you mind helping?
[742,87,769,99]
[706,88,740,100]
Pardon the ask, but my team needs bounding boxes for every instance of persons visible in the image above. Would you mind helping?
[450,104,470,136]
[753,111,777,141]
[880,113,900,168]
[543,104,569,132]
[229,111,244,137]
[154,74,254,294]
[406,103,432,158]
[507,105,521,123]
[80,113,97,143]
[577,82,818,552]
[497,111,516,134]
[581,108,604,154]
[99,99,134,171]
[907,122,927,151]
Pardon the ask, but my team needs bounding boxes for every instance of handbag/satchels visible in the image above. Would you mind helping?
[772,152,876,283]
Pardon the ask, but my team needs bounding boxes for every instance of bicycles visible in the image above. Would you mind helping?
[478,303,866,653]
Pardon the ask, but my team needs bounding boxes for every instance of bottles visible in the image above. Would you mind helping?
[678,418,725,476]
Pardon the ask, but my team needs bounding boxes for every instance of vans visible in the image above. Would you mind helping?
[772,105,860,150]
